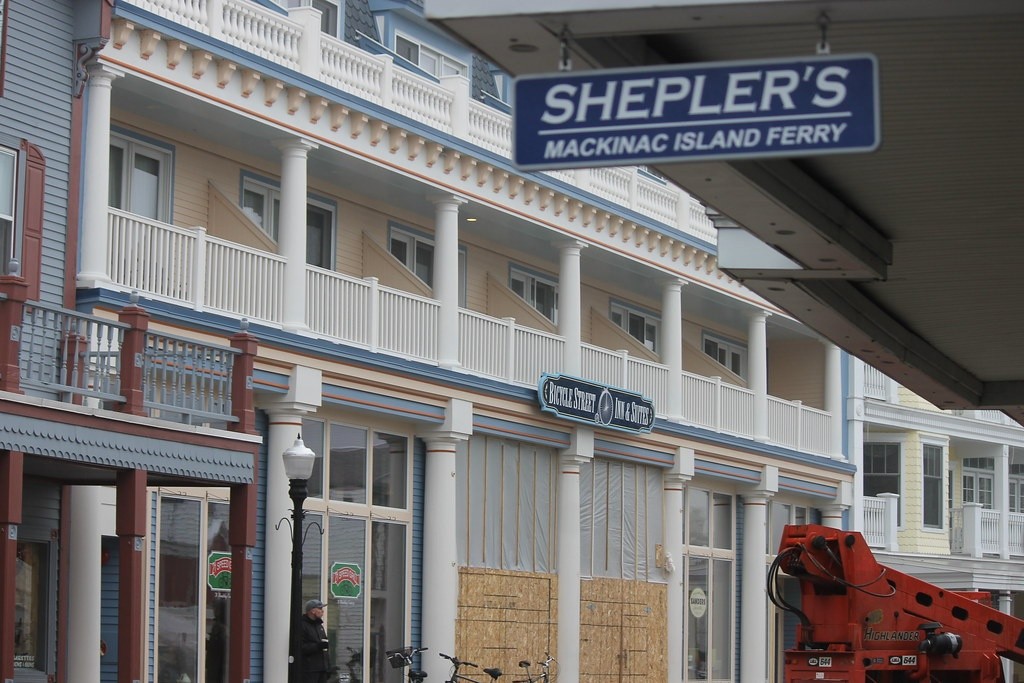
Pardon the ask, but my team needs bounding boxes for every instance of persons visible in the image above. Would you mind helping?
[290,599,333,683]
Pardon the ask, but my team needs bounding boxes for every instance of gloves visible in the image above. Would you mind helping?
[319,641,328,649]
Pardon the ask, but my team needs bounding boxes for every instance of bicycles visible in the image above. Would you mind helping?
[512,652,560,683]
[439,653,502,683]
[478,662,480,662]
[328,646,376,683]
[385,646,428,683]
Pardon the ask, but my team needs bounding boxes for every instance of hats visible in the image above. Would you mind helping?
[307,599,327,610]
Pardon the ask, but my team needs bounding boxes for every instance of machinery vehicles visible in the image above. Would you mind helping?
[767,524,1024,683]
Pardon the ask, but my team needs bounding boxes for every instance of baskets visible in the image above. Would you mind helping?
[386,646,414,668]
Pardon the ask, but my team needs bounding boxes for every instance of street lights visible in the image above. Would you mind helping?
[274,432,325,683]
